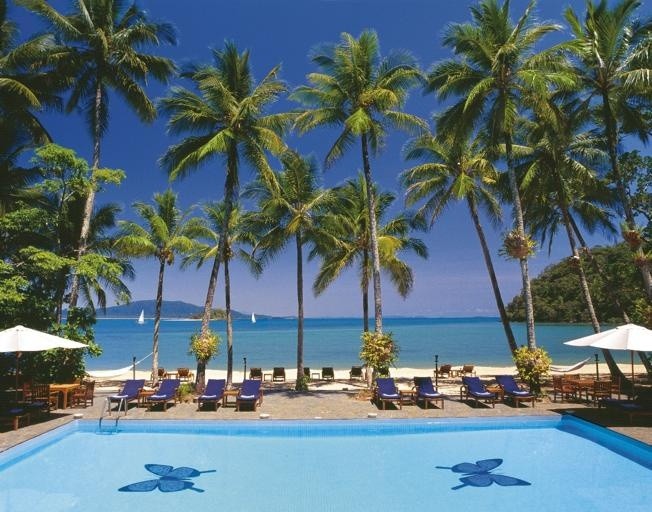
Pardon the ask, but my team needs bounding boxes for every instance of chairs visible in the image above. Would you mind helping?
[553,373,652,424]
[373,364,535,410]
[0,378,96,431]
[107,367,286,412]
[304,365,363,381]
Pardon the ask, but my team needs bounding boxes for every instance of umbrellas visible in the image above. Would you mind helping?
[0,324,90,403]
[563,322,652,401]
